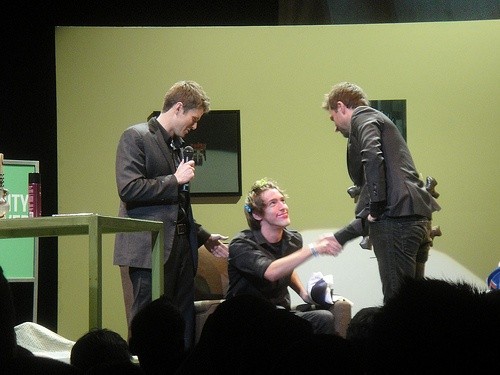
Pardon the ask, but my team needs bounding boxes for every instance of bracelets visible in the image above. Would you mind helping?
[310,243,320,257]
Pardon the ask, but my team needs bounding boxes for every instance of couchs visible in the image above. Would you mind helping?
[186,229,489,348]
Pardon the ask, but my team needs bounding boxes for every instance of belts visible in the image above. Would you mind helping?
[174,224,190,235]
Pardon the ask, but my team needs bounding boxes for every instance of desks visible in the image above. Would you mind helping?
[0,215,165,333]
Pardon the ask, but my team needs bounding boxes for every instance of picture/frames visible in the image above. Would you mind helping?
[366,98,407,144]
[147,110,241,198]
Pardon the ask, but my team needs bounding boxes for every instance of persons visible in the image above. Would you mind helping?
[315,81,441,308]
[112,81,228,354]
[228,179,343,336]
[0,267,500,375]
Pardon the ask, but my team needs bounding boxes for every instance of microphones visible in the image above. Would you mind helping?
[183,146,194,191]
